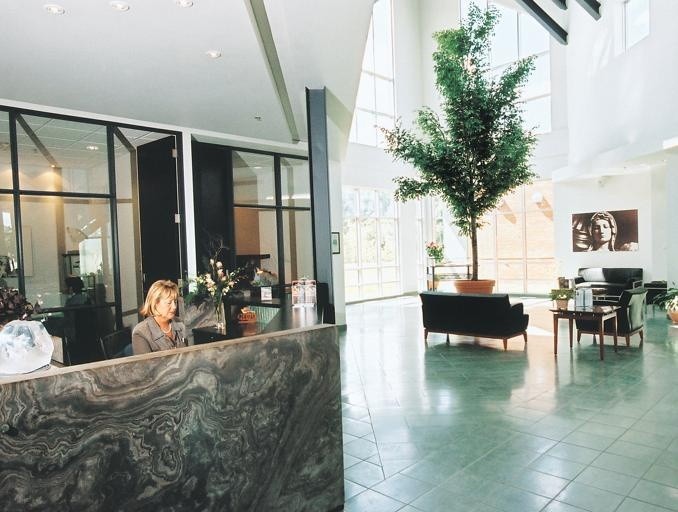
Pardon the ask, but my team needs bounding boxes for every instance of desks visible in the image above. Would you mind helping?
[549,304,621,360]
[192,321,281,345]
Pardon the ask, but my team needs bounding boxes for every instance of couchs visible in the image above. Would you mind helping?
[419,290,529,351]
[572,268,643,304]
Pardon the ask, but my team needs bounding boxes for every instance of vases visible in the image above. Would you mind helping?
[260,287,273,300]
[217,300,232,336]
[426,256,441,266]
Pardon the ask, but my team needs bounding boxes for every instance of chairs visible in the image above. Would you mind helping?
[94,326,134,359]
[575,286,650,348]
[53,306,115,366]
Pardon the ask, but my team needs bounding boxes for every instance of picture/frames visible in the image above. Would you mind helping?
[330,232,340,254]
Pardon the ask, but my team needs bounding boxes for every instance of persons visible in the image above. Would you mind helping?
[131,279,195,358]
[61,275,94,315]
[585,211,619,251]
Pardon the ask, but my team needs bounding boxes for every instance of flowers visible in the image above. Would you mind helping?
[250,266,280,286]
[178,233,248,313]
[423,240,444,263]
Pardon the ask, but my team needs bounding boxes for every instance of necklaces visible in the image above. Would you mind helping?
[161,323,173,334]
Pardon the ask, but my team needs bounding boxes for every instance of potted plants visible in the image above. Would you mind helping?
[651,288,678,325]
[549,288,574,310]
[373,1,547,295]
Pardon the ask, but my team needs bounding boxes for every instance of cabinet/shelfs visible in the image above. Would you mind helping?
[644,281,667,305]
[425,264,480,294]
[62,254,80,278]
